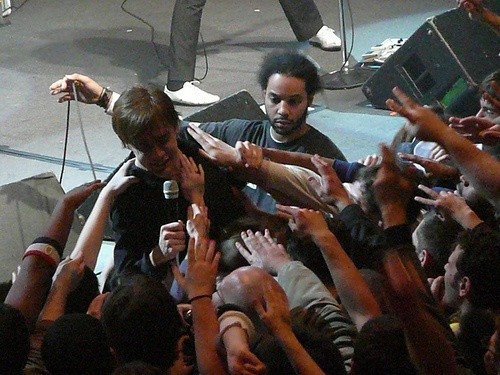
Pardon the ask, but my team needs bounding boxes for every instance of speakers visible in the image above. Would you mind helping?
[0,172,82,283]
[76,156,136,241]
[183,88,267,120]
[361,0,500,110]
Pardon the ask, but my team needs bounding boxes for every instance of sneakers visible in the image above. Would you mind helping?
[163,81,220,106]
[308,25,342,52]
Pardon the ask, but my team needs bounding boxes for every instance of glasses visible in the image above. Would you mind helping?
[215,277,225,305]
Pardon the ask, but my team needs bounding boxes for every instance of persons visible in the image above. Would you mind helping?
[99,85,238,301]
[361,1,500,119]
[156,0,334,106]
[48,50,349,269]
[0,71,499,375]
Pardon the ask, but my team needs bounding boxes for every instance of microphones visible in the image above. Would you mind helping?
[162,179,180,232]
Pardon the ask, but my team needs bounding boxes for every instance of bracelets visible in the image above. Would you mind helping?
[96,86,113,109]
